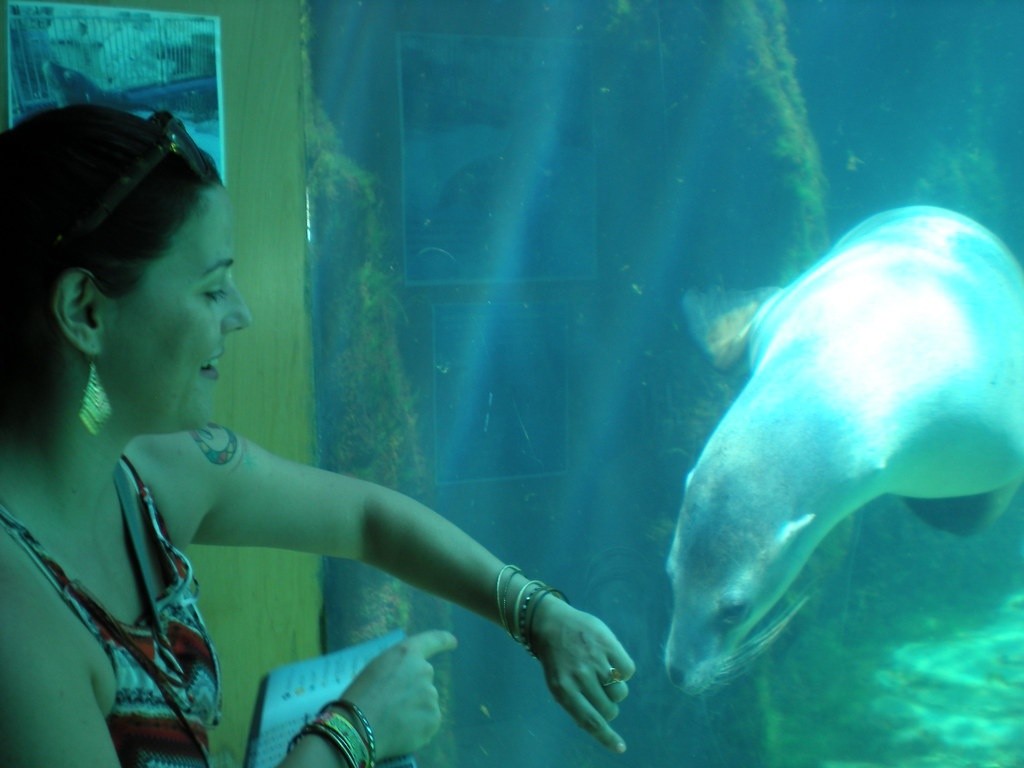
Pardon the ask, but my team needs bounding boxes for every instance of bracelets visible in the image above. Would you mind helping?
[494,561,575,659]
[285,697,378,768]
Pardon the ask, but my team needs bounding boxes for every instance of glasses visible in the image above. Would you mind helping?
[53,109,206,252]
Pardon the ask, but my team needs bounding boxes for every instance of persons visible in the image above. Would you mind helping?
[3,104,633,763]
